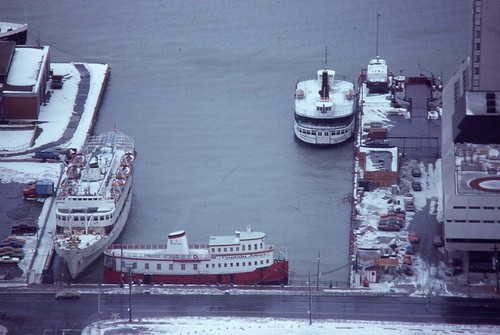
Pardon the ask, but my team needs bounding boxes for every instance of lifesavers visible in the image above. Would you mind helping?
[193,255,197,259]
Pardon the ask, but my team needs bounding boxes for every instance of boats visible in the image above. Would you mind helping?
[53,124,136,277]
[347,69,445,295]
[294,46,356,146]
[104,226,289,286]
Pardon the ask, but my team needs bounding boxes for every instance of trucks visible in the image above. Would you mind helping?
[23,181,54,195]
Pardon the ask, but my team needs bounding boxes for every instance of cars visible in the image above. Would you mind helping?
[0,248,23,253]
[10,221,38,231]
[0,251,24,259]
[3,236,27,246]
[0,256,19,263]
